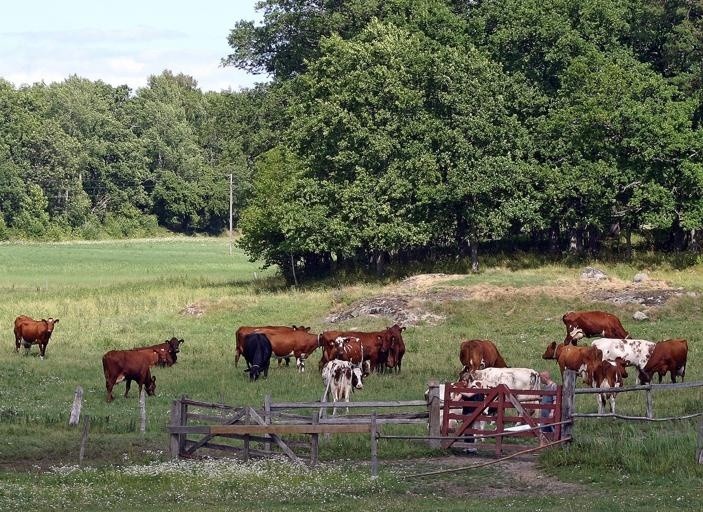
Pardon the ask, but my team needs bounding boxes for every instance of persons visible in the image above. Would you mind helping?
[536,371,558,451]
[451,371,486,454]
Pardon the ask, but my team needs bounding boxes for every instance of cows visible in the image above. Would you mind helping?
[322,359,369,415]
[542,310,688,421]
[235,324,406,381]
[103,337,184,403]
[460,340,508,371]
[424,366,542,443]
[14,315,59,360]
